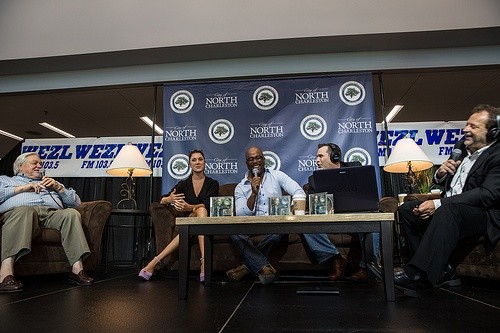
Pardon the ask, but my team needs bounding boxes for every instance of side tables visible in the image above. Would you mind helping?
[97,209,152,276]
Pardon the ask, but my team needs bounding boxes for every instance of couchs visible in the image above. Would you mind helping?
[150,182,398,276]
[14,200,112,279]
[403,192,500,281]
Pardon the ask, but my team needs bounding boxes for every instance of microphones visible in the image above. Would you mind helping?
[252,169,259,178]
[39,169,50,189]
[446,149,462,180]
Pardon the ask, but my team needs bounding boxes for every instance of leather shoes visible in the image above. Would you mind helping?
[366,262,425,298]
[69,270,94,285]
[433,268,461,289]
[329,253,348,280]
[0,275,24,292]
[351,268,367,279]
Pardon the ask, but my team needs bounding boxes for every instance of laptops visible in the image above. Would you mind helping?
[313,166,381,213]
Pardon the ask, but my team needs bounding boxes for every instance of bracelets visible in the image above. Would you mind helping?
[58,185,64,191]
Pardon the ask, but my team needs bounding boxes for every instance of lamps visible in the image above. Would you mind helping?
[384,137,435,194]
[106,141,153,210]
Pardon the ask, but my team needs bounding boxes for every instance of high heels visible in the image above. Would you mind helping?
[200,258,204,281]
[138,256,161,281]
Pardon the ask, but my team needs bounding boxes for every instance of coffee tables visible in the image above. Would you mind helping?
[175,212,397,303]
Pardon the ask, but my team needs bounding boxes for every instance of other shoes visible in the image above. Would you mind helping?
[225,265,249,280]
[259,264,278,282]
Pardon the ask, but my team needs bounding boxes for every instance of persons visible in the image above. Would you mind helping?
[367,104,500,297]
[224,146,306,286]
[139,150,221,281]
[0,152,94,292]
[299,143,370,282]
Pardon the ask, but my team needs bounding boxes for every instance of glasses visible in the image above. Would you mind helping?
[190,149,202,153]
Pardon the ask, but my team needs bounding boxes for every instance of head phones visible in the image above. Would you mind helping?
[329,143,341,163]
[487,116,500,141]
